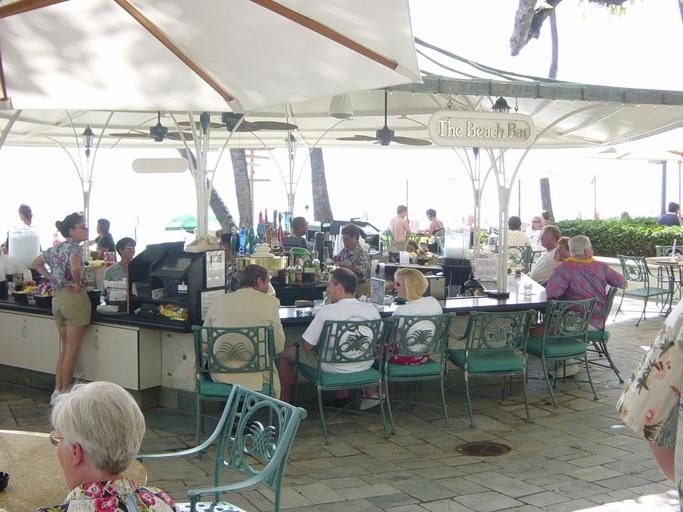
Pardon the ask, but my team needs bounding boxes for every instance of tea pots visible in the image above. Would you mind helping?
[254,243,270,254]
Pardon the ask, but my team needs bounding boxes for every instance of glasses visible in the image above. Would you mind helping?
[49,430,65,446]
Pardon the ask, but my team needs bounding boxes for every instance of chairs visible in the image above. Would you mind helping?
[185,324,276,443]
[290,317,390,446]
[132,383,313,512]
[517,297,600,408]
[613,242,683,327]
[560,287,624,385]
[446,308,536,428]
[507,245,532,276]
[352,313,450,434]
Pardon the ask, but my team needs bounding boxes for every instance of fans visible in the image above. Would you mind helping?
[334,91,434,149]
[109,114,298,143]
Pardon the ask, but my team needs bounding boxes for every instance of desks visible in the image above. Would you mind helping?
[0,429,145,512]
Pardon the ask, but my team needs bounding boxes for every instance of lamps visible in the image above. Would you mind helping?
[328,93,353,121]
[489,97,511,112]
[79,123,96,160]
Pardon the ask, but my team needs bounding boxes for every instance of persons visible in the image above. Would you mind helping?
[88,219,113,259]
[507,203,628,378]
[34,380,185,512]
[281,217,307,252]
[390,205,411,243]
[419,210,445,252]
[33,213,91,406]
[332,225,371,283]
[277,267,383,404]
[104,238,136,281]
[202,264,285,400]
[3,204,41,268]
[655,201,683,228]
[359,267,442,411]
[614,294,683,511]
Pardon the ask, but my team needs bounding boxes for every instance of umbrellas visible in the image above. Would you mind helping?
[0,0,425,116]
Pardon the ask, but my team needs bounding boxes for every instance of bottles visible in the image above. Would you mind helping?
[248,225,254,253]
[238,225,246,255]
[230,227,235,249]
[285,251,320,285]
[329,226,344,255]
[381,232,420,252]
[257,208,284,254]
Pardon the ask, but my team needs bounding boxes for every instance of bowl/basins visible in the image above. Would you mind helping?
[436,258,471,278]
[33,295,52,306]
[11,292,26,302]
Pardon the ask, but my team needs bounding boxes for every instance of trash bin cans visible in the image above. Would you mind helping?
[164,215,198,233]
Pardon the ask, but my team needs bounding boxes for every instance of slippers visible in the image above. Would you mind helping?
[327,395,351,407]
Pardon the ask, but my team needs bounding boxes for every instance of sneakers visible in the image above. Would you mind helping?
[359,392,385,410]
[549,365,580,378]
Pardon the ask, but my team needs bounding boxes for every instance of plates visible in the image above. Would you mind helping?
[249,254,273,258]
[97,302,125,316]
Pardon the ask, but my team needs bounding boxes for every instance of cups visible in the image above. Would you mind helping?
[523,283,532,294]
[515,268,520,274]
[324,240,334,257]
[322,291,331,304]
[322,247,329,260]
[399,251,409,265]
